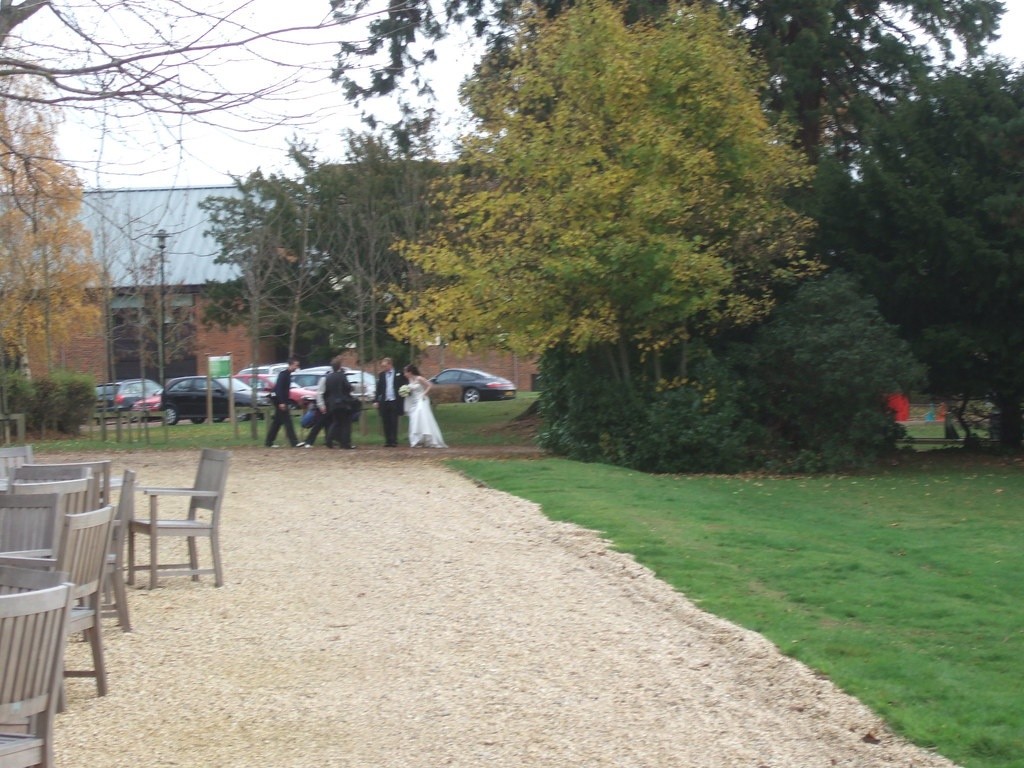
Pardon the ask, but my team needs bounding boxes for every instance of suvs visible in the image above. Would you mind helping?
[237,362,304,384]
[287,365,378,403]
[94,379,162,425]
[159,375,273,426]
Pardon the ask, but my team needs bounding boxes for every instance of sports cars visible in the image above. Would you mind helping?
[424,368,518,404]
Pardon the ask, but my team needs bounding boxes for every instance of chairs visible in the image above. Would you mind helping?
[0,443,230,768]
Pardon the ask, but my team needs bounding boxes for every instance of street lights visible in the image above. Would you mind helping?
[151,229,173,386]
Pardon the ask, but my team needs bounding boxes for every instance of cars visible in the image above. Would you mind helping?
[129,388,164,420]
[231,374,319,412]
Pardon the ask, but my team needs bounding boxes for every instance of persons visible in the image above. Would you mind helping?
[403,363,434,448]
[372,357,410,448]
[264,355,306,448]
[304,357,357,449]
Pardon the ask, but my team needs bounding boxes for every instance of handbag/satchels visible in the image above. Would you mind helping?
[300,408,321,428]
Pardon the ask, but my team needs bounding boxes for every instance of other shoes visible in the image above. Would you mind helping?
[271,445,280,447]
[351,445,357,449]
[305,444,313,448]
[295,442,305,446]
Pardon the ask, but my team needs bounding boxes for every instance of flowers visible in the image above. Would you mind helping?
[398,384,410,398]
